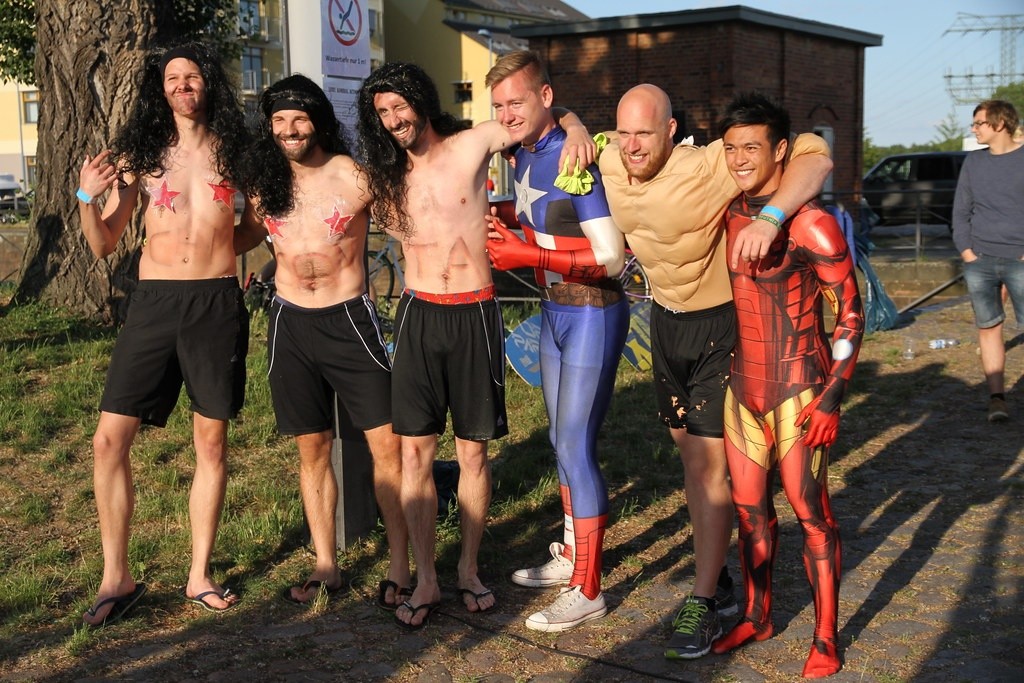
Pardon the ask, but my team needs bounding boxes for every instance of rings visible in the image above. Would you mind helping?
[84,160,89,165]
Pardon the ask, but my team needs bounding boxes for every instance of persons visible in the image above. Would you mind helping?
[952,99,1024,421]
[75,41,866,678]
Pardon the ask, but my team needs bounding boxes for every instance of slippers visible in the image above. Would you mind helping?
[281,579,333,608]
[179,586,242,614]
[394,600,441,631]
[456,586,499,615]
[378,579,413,612]
[81,582,148,630]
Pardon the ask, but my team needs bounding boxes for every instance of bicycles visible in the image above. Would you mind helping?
[367,231,404,301]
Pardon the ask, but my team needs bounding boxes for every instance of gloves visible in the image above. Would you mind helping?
[794,387,842,450]
[485,219,539,272]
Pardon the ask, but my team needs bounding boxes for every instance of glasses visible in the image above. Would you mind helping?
[970,119,987,127]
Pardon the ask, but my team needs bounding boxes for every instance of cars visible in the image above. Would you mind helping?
[862,151,969,234]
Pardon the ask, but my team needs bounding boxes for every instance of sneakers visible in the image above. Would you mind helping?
[511,542,575,587]
[663,589,723,660]
[526,584,608,633]
[713,576,739,618]
[988,397,1009,422]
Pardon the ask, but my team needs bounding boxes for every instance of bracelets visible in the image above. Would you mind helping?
[759,205,786,224]
[76,188,98,205]
[756,214,781,231]
